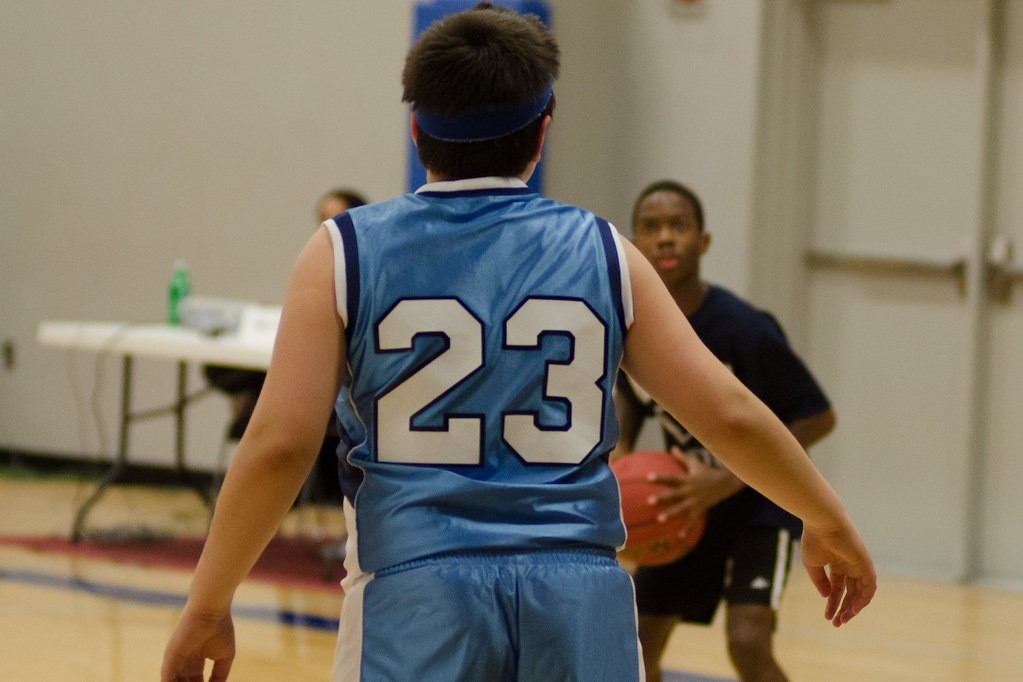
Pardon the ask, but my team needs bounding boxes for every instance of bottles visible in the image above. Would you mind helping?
[167,259,189,326]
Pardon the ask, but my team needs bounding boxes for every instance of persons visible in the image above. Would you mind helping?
[608,180,838,682]
[258,189,370,550]
[161,0,877,682]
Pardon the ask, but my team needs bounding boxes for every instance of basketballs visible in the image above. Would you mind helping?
[606,451,704,565]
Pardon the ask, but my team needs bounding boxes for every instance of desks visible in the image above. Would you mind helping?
[40,315,342,546]
